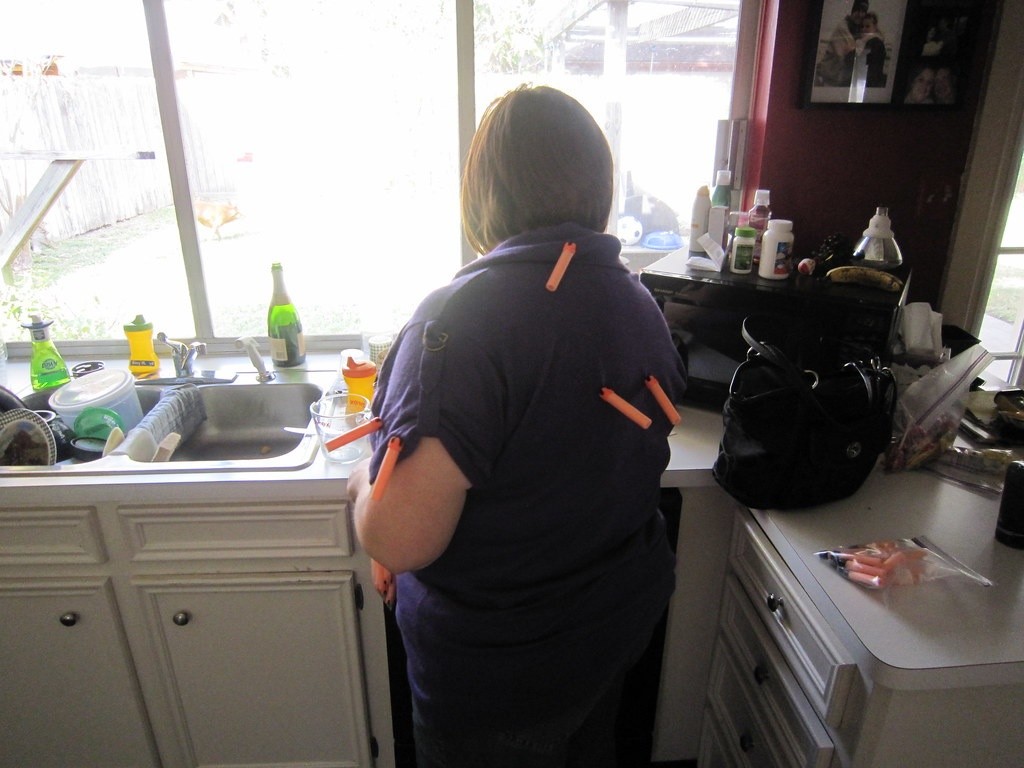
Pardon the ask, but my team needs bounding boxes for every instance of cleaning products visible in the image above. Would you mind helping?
[19,313,71,392]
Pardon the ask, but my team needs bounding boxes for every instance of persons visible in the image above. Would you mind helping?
[345,86,687,768]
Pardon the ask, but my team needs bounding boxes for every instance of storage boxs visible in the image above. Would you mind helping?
[708,206,731,249]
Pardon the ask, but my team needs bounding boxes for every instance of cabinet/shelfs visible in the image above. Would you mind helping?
[1,500,394,768]
[697,503,1024,768]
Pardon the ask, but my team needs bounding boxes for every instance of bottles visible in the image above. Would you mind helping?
[689,171,795,279]
[123,314,161,372]
[268,262,305,367]
[342,356,376,411]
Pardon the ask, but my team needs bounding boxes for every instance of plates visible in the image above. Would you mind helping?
[0,408,56,466]
[102,428,125,457]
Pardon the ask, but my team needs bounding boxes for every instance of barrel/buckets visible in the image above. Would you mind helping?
[48,369,143,436]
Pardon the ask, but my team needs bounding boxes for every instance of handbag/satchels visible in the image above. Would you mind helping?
[712,311,898,513]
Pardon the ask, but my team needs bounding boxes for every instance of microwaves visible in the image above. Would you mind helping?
[640,246,914,410]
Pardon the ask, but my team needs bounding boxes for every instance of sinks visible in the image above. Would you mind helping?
[0,380,165,477]
[127,383,320,475]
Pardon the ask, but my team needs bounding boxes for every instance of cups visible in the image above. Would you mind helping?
[310,393,375,464]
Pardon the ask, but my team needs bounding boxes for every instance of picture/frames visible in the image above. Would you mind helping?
[801,0,985,111]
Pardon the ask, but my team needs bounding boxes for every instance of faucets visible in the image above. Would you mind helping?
[234,337,275,382]
[158,332,208,379]
